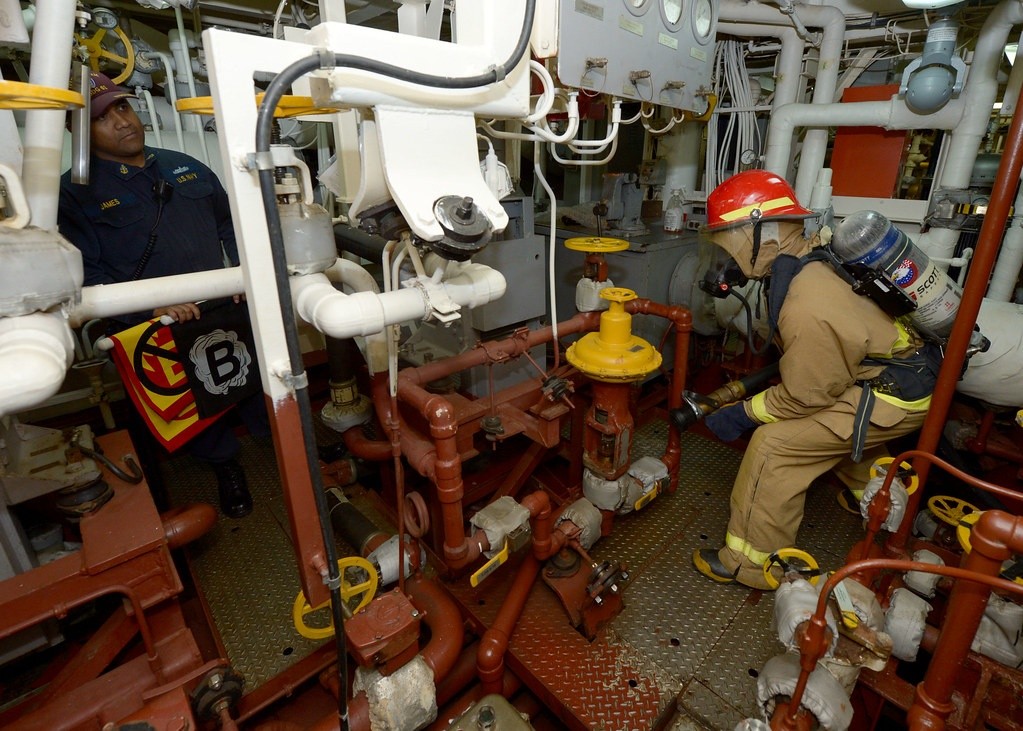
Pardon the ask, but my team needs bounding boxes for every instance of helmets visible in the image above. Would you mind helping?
[698,168,822,235]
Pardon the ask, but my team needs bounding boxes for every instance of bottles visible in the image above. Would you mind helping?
[664,191,684,230]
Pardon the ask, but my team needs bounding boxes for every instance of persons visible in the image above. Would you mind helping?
[58,70,256,517]
[690,169,933,590]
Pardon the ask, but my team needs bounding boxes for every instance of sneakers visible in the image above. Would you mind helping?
[837,490,862,515]
[693,548,744,586]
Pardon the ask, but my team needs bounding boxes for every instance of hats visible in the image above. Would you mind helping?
[66,70,139,126]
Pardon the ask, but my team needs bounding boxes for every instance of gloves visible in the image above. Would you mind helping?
[706,398,757,443]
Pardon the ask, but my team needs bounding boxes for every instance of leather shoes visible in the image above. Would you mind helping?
[214,462,252,518]
[316,441,348,464]
